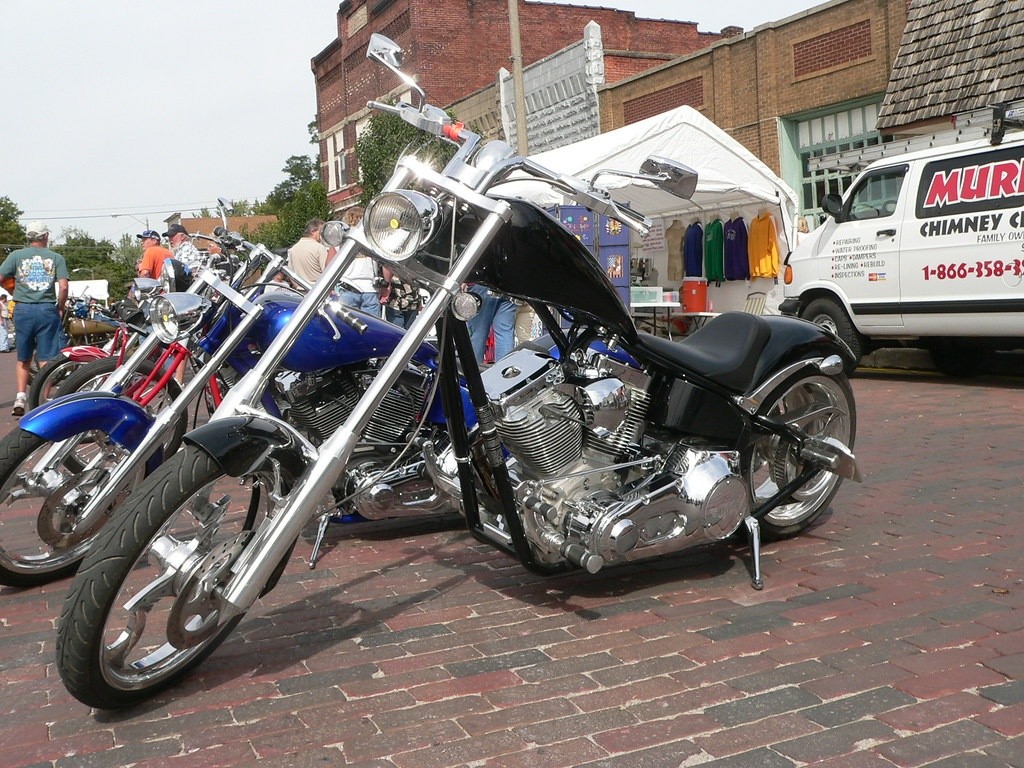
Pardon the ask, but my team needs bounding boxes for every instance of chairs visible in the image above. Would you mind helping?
[743,292,769,316]
[631,286,667,336]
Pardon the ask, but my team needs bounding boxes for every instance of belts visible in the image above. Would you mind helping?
[16,301,55,304]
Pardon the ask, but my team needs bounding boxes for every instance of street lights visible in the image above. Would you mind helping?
[111,212,150,232]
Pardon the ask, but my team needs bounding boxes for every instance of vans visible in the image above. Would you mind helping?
[773,132,1024,376]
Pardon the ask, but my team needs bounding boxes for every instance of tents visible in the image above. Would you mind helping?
[0,279,110,309]
[489,104,798,313]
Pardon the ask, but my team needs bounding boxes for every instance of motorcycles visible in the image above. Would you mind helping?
[55,43,862,712]
[0,199,643,589]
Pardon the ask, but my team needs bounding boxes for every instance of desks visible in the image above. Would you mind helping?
[672,311,722,334]
[630,302,680,341]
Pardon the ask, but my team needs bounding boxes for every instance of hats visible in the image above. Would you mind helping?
[26,221,52,238]
[162,223,188,236]
[136,230,161,241]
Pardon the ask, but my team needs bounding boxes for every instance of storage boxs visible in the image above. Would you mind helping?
[663,291,680,303]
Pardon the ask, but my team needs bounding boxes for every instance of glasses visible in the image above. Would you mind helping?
[209,243,214,247]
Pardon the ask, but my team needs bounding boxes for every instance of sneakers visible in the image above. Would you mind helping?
[11,397,25,417]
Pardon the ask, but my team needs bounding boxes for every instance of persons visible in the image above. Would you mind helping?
[796,216,810,246]
[664,207,779,282]
[0,206,543,414]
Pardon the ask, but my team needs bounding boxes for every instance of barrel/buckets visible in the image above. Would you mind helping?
[682,277,708,313]
